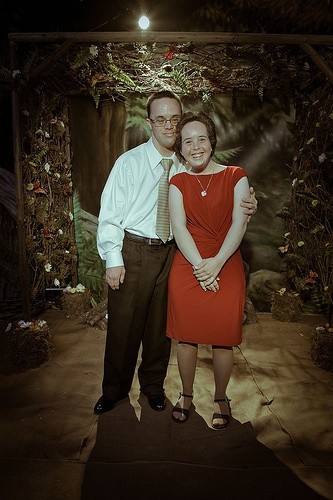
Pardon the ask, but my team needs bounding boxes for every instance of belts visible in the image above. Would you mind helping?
[124,230,173,245]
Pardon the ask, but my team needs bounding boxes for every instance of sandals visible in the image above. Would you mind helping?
[172,392,194,423]
[212,396,232,429]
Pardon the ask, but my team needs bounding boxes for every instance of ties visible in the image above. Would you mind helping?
[156,158,174,244]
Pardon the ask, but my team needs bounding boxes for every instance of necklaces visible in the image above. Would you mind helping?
[194,166,215,196]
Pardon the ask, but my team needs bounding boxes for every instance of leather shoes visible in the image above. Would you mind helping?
[94,393,129,415]
[146,386,168,412]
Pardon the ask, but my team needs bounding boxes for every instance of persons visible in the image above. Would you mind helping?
[166,111,250,429]
[94,90,258,413]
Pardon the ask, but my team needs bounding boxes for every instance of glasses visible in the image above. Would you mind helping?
[149,117,180,127]
